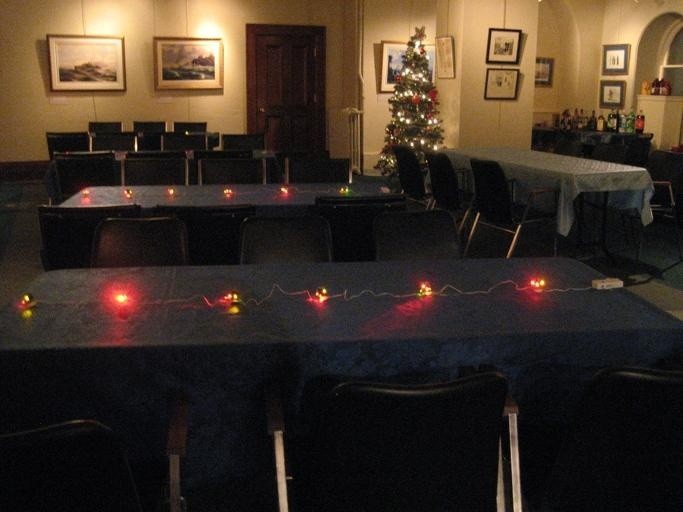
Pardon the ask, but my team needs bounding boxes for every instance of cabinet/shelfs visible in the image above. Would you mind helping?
[635,93,683,160]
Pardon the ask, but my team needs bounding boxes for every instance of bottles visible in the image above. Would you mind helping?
[552,107,645,133]
[640,78,670,96]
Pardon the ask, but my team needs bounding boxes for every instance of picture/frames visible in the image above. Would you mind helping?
[151,35,226,92]
[379,27,630,109]
[45,33,126,95]
